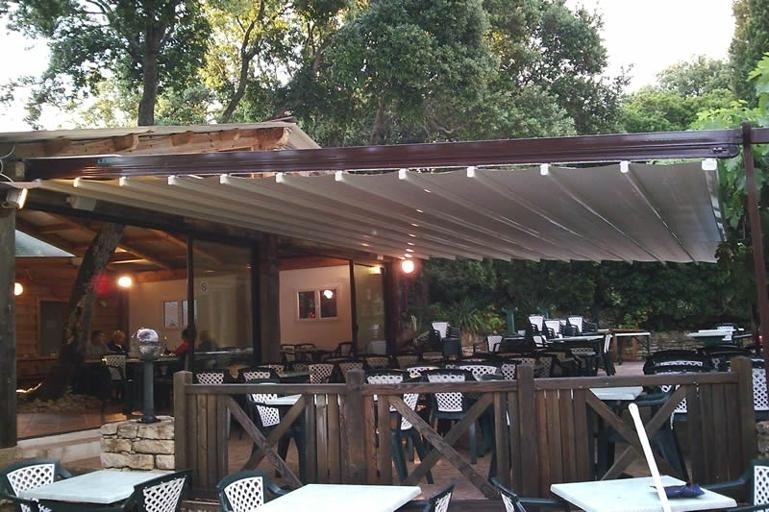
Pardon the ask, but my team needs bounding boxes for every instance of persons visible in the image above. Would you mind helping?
[198,330,222,352]
[107,329,127,353]
[171,329,197,355]
[89,330,110,361]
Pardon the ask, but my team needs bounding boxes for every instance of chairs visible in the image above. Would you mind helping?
[216,468,289,512]
[238,366,280,384]
[710,349,750,371]
[716,317,756,354]
[264,362,285,378]
[400,483,456,512]
[247,378,290,477]
[711,464,769,512]
[431,321,465,365]
[491,478,571,512]
[282,340,357,361]
[285,362,306,372]
[561,315,583,336]
[643,349,713,429]
[125,468,193,512]
[335,362,366,383]
[0,458,73,512]
[722,358,769,417]
[191,368,237,385]
[517,310,563,354]
[366,348,599,486]
[306,363,336,384]
[486,335,504,356]
[100,352,136,419]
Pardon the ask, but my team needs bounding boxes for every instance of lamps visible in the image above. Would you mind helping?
[397,258,416,276]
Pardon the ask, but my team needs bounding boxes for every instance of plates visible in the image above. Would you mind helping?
[649,482,704,499]
